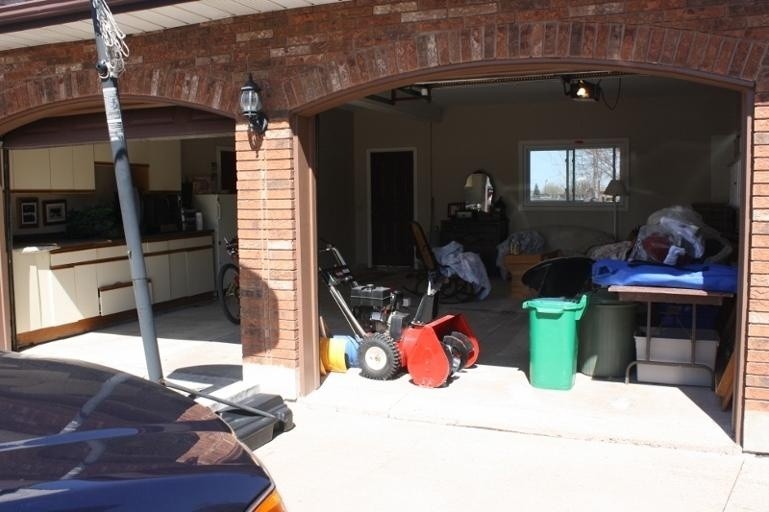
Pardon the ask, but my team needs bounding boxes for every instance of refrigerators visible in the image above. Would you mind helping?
[193,189,238,295]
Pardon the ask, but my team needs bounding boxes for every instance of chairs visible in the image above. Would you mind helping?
[403,222,486,305]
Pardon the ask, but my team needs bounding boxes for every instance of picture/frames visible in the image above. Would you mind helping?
[16,197,69,229]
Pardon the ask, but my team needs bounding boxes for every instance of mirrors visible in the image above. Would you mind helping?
[463,171,494,215]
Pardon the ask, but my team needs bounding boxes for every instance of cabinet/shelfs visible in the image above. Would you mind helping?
[440,218,510,279]
[2,140,183,193]
[10,235,219,351]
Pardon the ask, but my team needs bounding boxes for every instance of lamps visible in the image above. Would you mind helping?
[238,71,269,134]
[563,75,603,102]
[603,179,629,242]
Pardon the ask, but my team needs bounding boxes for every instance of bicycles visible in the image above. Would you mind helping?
[217,235,242,325]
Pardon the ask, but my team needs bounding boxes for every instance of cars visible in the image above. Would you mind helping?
[0,349,288,512]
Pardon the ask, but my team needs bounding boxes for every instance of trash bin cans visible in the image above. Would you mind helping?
[522,291,591,390]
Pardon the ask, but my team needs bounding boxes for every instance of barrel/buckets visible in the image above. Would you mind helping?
[577,289,641,381]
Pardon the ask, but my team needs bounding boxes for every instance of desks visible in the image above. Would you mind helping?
[503,249,560,297]
[606,285,735,391]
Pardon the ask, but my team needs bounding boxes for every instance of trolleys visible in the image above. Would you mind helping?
[313,236,481,389]
